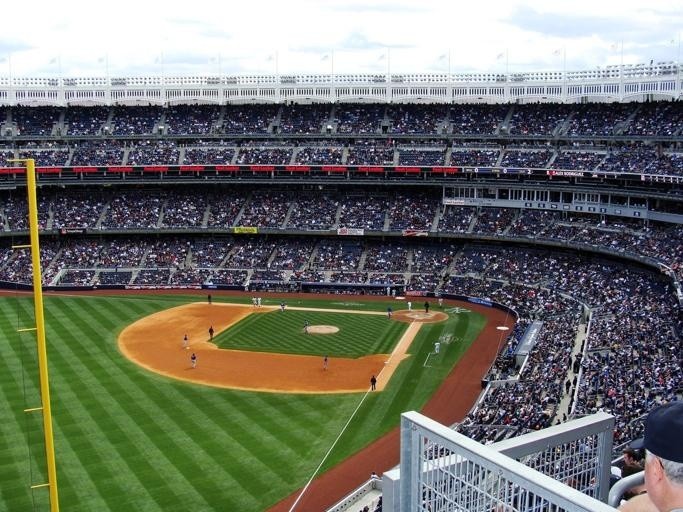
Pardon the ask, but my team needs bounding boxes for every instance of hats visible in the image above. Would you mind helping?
[629,401,683,463]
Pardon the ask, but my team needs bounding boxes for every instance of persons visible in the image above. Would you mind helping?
[0,100,683,512]
